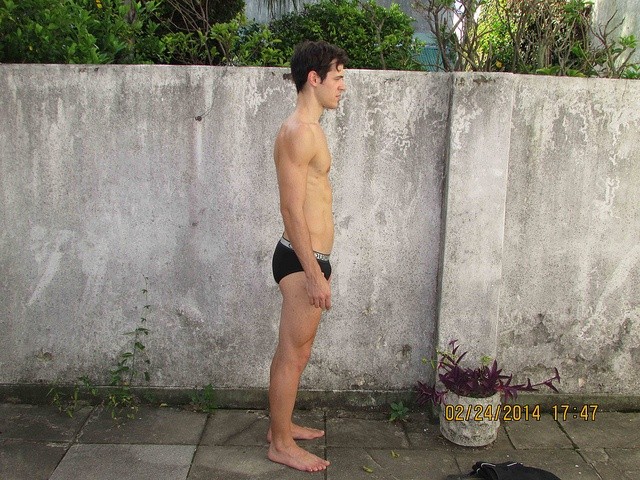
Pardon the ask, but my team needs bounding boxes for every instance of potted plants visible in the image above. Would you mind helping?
[419,338,561,448]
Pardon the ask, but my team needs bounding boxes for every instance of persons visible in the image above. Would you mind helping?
[267,41,346,472]
